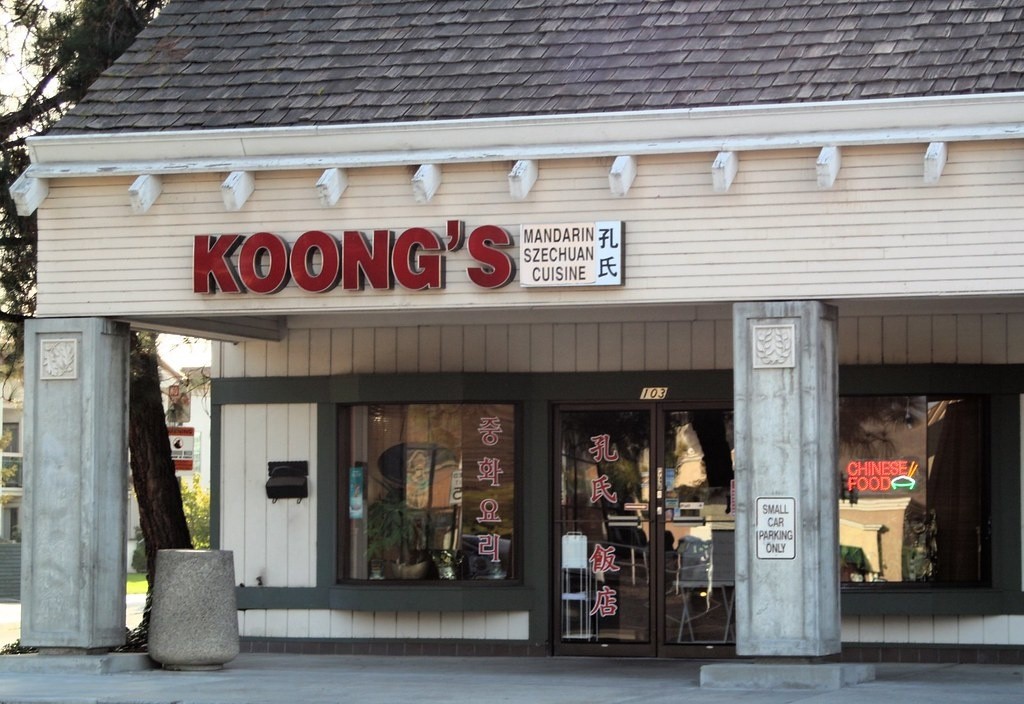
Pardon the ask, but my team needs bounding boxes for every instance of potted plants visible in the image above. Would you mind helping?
[364,489,431,579]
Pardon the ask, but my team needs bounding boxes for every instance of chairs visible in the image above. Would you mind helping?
[679,531,736,644]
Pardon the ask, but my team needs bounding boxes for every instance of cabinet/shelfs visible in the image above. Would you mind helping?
[561,558,599,643]
